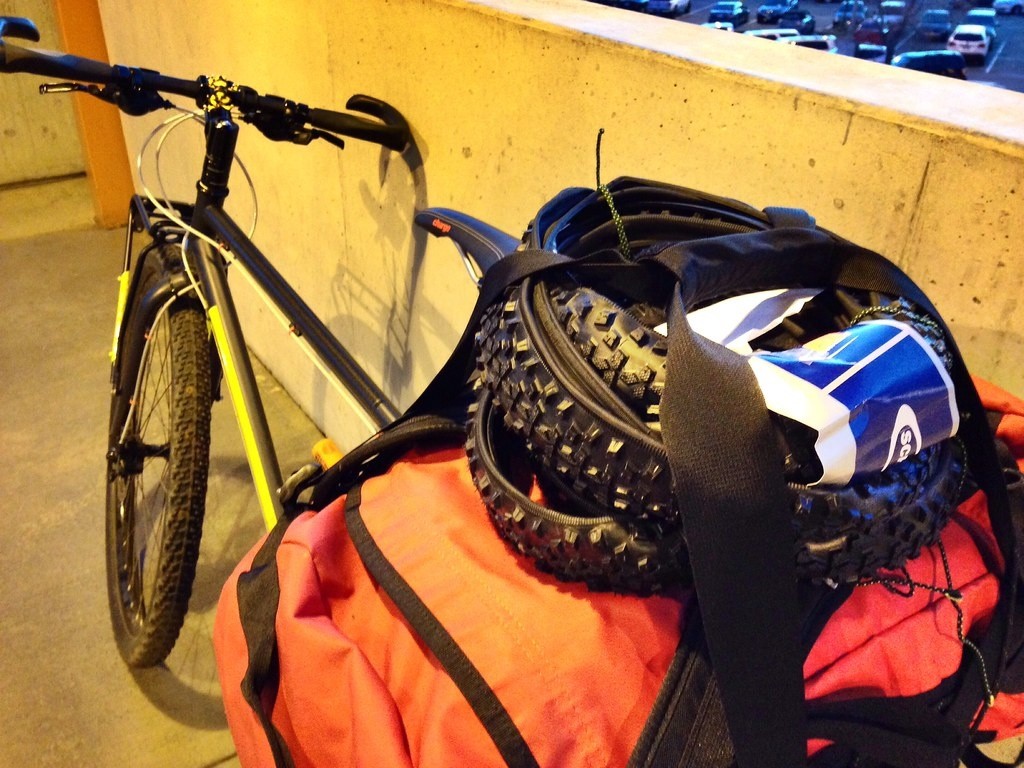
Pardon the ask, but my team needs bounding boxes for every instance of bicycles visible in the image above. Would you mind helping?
[1,13,524,672]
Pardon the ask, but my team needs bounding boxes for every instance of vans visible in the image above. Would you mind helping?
[891,49,966,81]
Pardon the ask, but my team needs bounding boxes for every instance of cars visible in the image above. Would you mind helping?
[959,7,998,42]
[945,25,991,66]
[914,9,952,43]
[991,0,1024,15]
[606,0,909,63]
